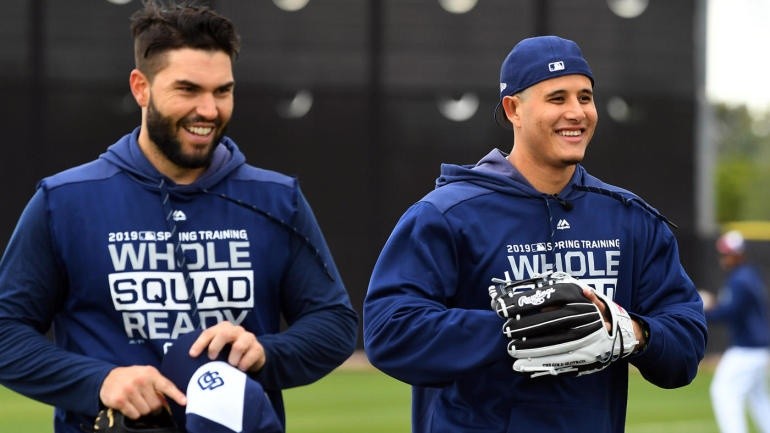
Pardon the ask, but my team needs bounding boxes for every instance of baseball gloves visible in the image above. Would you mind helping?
[492,273,637,379]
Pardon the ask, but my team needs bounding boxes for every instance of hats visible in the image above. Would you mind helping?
[717,230,745,255]
[160,328,283,433]
[495,36,594,128]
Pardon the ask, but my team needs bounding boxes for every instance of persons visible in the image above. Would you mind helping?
[0,0,360,433]
[702,230,770,433]
[360,34,706,433]
